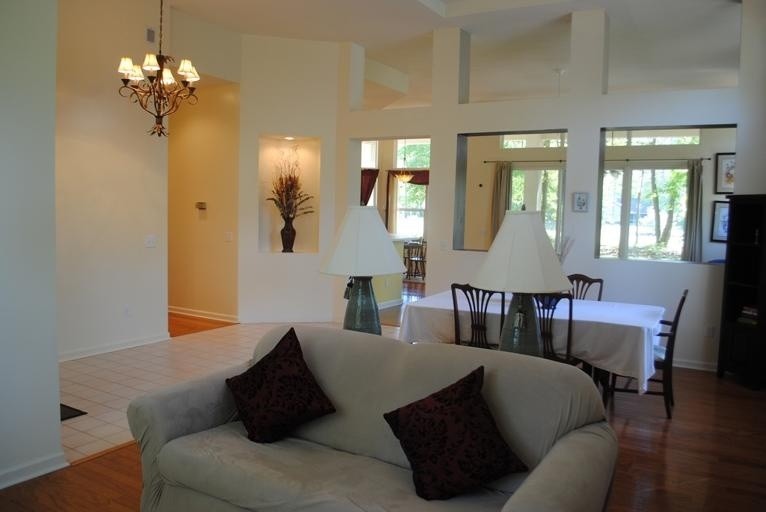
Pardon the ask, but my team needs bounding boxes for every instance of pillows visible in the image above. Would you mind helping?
[225,327,337,443]
[382,365,529,501]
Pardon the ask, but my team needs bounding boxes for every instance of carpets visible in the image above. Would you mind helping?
[61,403,87,422]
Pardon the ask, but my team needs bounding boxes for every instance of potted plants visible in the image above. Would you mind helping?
[263,148,316,253]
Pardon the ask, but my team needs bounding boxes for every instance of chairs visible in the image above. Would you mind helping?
[403,237,428,280]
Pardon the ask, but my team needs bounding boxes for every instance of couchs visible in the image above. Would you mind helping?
[126,326,620,511]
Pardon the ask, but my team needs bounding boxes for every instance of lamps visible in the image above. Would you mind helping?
[393,138,415,185]
[117,0,201,138]
[468,205,574,357]
[318,201,408,334]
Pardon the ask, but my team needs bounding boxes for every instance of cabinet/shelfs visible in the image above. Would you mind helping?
[715,195,766,389]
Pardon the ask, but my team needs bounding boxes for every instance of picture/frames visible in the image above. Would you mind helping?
[713,152,736,195]
[710,200,731,242]
